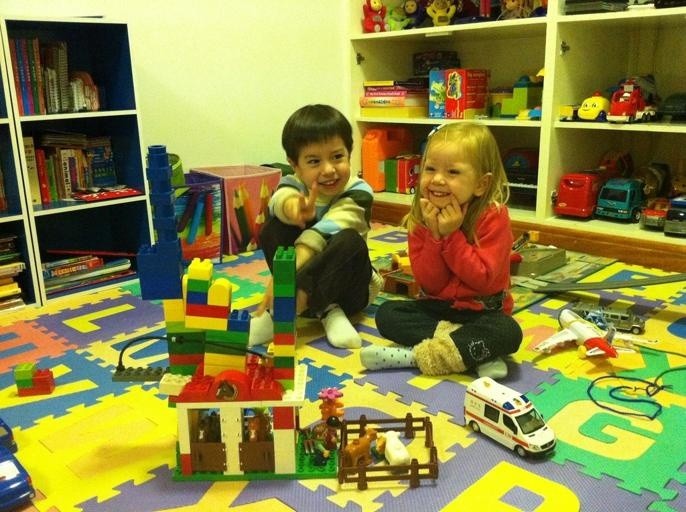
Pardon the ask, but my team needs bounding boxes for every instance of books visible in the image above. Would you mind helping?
[0,30,136,311]
[359,77,429,120]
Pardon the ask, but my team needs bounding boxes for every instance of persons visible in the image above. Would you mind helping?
[359,121,523,380]
[256,103,385,350]
[361,0,546,37]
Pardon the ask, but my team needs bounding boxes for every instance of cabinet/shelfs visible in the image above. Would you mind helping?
[351,0,686,272]
[1,18,155,311]
